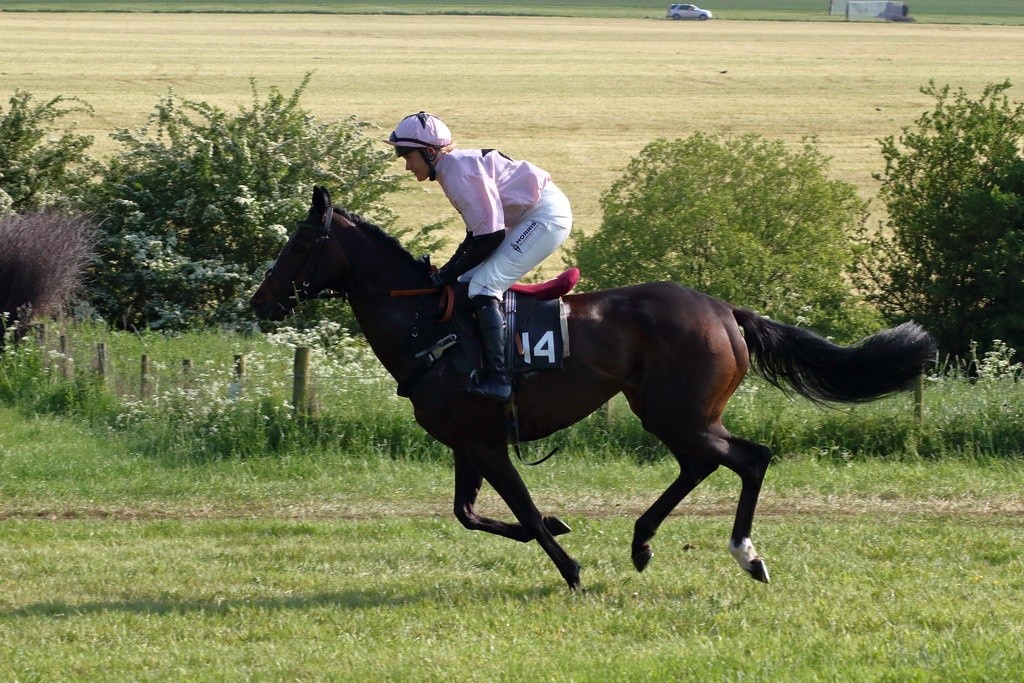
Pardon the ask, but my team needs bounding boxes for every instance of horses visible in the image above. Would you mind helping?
[249,185,939,590]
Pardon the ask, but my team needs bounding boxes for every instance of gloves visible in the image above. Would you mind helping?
[430,270,450,288]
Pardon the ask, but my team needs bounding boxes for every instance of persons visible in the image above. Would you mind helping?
[381,111,573,403]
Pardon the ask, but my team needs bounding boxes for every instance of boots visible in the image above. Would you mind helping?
[468,297,512,399]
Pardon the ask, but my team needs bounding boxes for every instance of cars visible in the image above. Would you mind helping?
[665,3,712,21]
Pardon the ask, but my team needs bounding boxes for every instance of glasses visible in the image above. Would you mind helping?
[389,131,431,146]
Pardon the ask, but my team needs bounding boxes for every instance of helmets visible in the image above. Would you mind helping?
[386,112,451,158]
